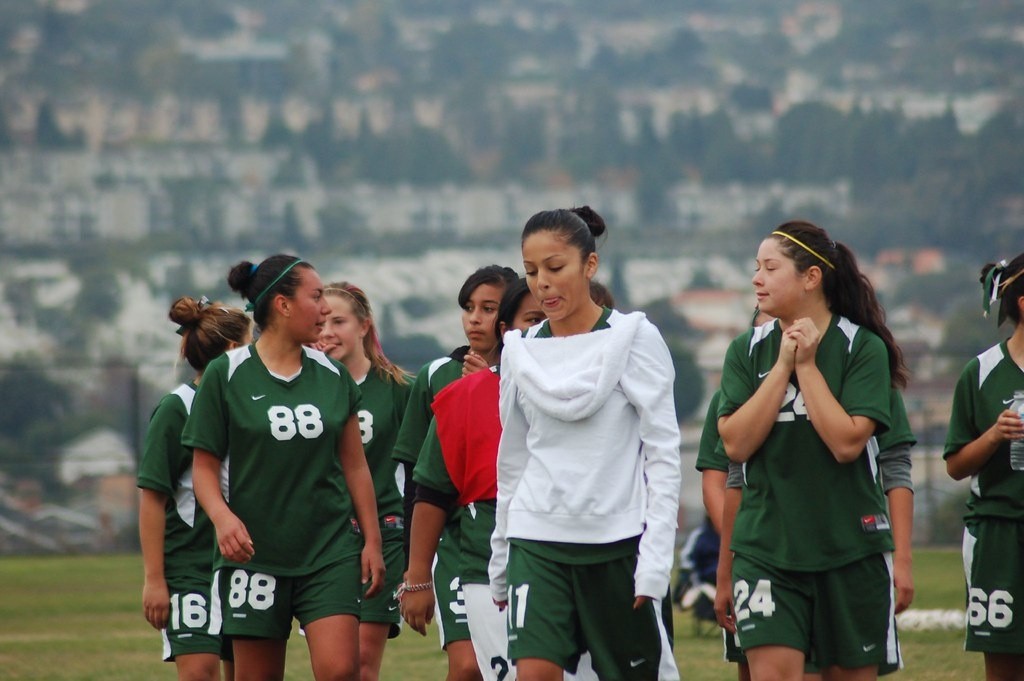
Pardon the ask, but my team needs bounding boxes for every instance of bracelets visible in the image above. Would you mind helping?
[393,582,433,599]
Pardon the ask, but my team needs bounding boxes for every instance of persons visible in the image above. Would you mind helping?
[302,283,417,681]
[392,264,616,681]
[488,206,682,681]
[136,294,256,681]
[180,255,385,681]
[942,246,1024,681]
[673,219,918,681]
